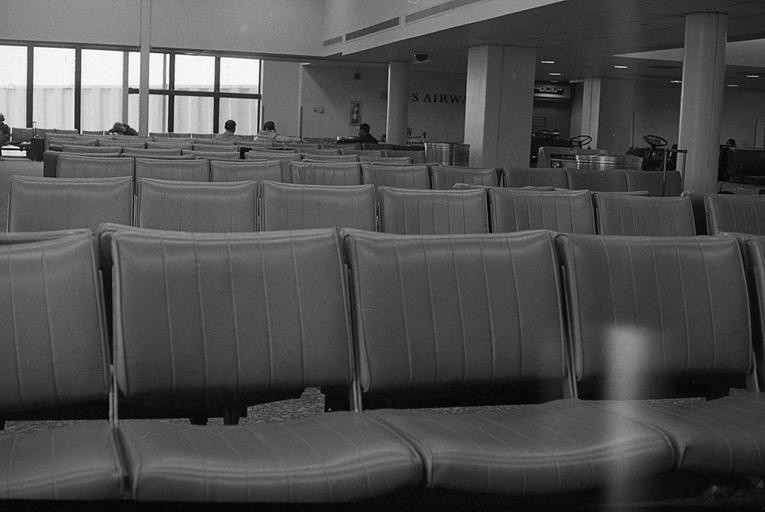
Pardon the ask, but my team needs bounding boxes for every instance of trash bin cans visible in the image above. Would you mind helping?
[575,154,643,172]
[31,136,44,162]
[425,142,471,172]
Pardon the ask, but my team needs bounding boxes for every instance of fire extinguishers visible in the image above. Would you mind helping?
[353,105,358,123]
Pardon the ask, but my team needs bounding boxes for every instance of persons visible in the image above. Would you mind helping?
[725,138,738,147]
[336,123,378,143]
[214,120,243,141]
[109,122,138,136]
[255,121,308,143]
[0,112,11,146]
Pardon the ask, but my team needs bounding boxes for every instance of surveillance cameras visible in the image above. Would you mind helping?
[415,53,429,62]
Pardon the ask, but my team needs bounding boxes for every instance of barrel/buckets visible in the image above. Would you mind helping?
[573,152,644,171]
[422,140,471,168]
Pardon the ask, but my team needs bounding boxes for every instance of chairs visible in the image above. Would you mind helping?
[3,131,763,230]
[344,231,677,510]
[0,236,126,512]
[114,229,421,509]
[559,228,765,512]
